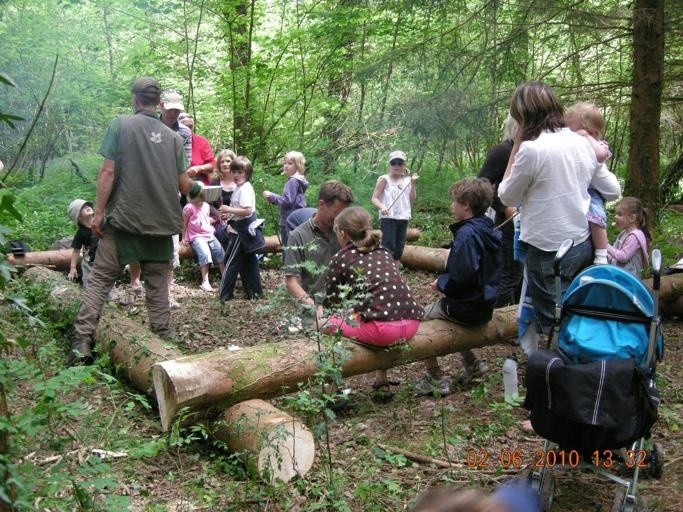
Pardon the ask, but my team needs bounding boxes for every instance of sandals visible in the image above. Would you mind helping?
[371,379,393,401]
[325,390,348,410]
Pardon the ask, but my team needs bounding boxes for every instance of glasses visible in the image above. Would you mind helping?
[390,160,404,166]
[178,114,193,120]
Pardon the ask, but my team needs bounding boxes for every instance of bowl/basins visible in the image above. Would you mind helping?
[201,186,224,202]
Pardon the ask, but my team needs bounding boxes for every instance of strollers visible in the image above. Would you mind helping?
[516,233,669,512]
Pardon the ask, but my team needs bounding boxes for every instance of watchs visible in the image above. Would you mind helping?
[211,148,236,242]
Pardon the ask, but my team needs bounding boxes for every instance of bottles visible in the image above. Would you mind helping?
[502,354,517,404]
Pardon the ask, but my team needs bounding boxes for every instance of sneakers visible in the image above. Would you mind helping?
[460,359,486,384]
[416,375,452,395]
[64,341,94,367]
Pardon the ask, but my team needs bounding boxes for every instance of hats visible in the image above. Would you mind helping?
[389,151,407,162]
[160,89,184,110]
[68,199,93,225]
[132,78,161,94]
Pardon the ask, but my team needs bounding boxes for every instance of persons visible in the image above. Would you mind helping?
[284,207,318,241]
[497,82,620,435]
[409,456,648,511]
[178,113,217,186]
[262,150,309,260]
[284,180,356,313]
[411,177,503,398]
[65,196,94,283]
[159,87,193,163]
[310,204,424,347]
[608,196,650,279]
[370,150,420,272]
[479,113,525,306]
[180,178,225,294]
[59,75,205,371]
[563,102,611,262]
[215,155,265,299]
